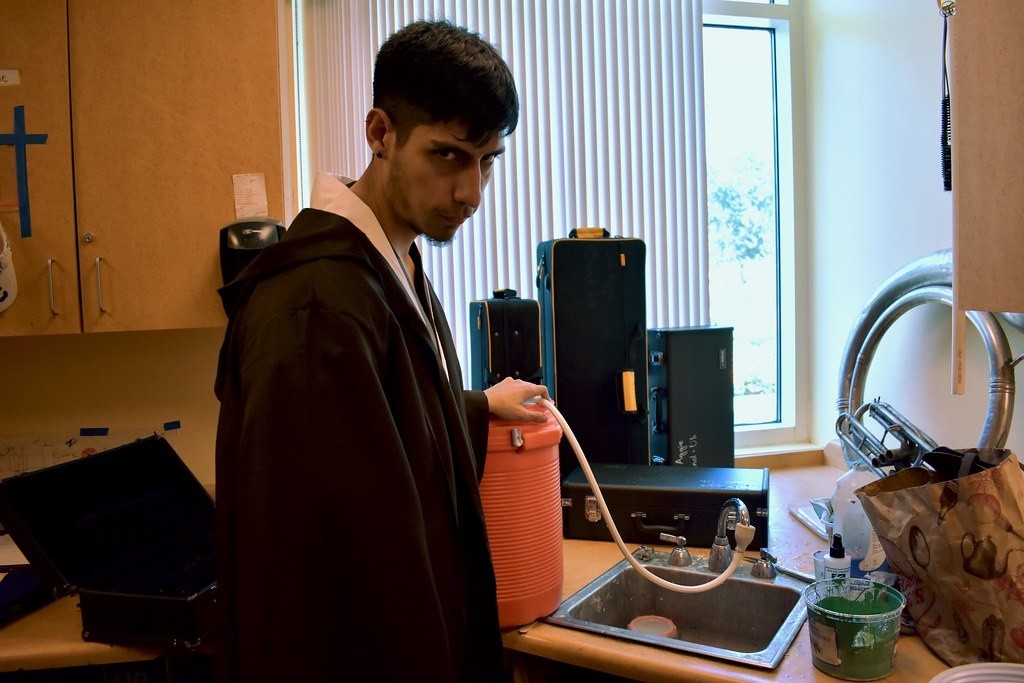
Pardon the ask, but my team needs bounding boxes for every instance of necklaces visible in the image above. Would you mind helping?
[376,223,442,370]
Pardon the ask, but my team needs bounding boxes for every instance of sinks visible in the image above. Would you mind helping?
[539,544,814,671]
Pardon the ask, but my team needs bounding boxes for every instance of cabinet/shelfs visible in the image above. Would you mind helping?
[0,0,300,337]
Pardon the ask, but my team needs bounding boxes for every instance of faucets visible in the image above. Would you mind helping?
[708,497,750,574]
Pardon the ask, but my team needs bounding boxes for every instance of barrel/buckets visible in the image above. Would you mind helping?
[812,551,830,581]
[928,662,1024,683]
[477,394,564,629]
[803,578,907,681]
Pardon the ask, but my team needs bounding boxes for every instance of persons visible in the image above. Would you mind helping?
[213,21,520,683]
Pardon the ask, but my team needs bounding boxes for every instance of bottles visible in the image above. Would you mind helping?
[824,534,851,579]
